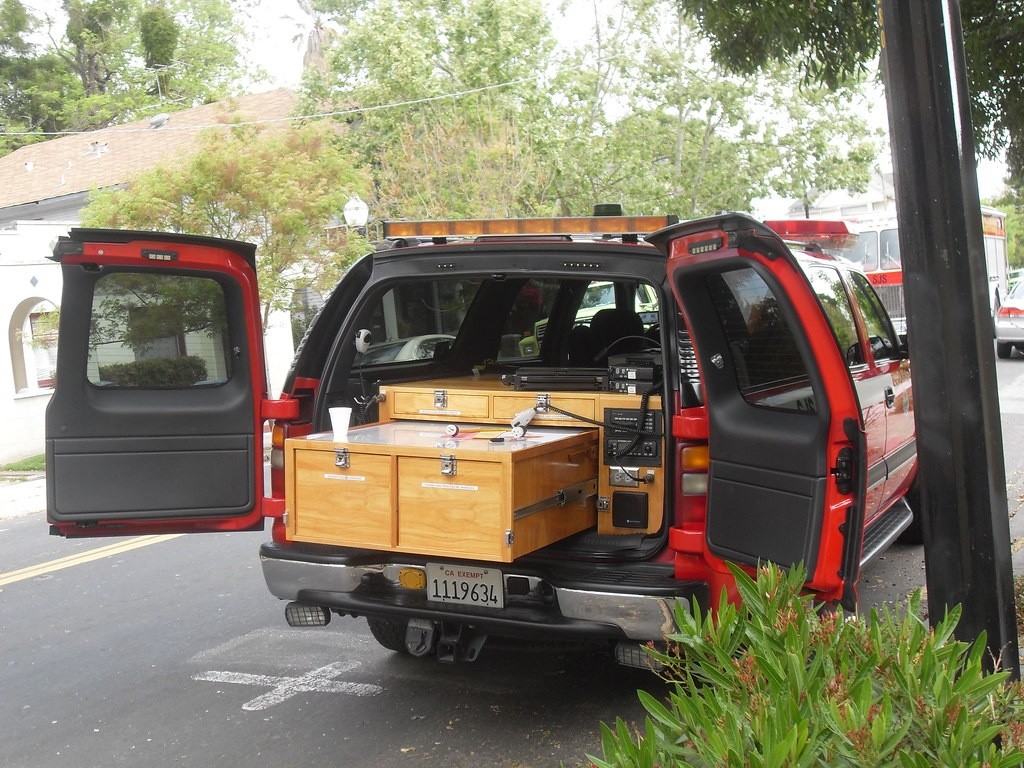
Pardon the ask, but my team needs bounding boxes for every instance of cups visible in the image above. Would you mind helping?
[328,406,352,441]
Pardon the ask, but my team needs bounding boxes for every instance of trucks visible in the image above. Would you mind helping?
[823,207,1009,355]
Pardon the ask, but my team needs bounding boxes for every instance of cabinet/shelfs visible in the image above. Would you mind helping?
[378,373,665,536]
[283,420,598,563]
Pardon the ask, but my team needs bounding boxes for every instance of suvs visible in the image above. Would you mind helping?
[45,205,924,673]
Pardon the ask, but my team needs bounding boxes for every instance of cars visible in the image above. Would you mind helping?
[994,281,1024,359]
